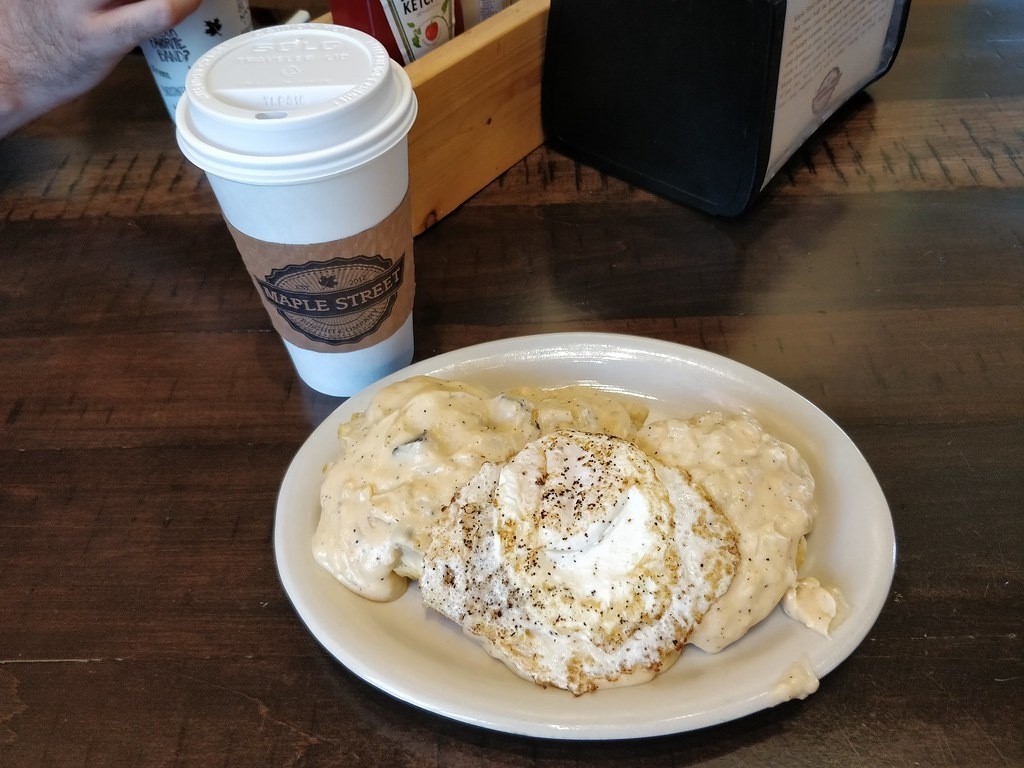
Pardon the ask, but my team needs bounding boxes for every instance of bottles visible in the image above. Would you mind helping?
[329,0,456,67]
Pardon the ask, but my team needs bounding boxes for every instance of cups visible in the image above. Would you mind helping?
[176,23,419,396]
[138,0,253,126]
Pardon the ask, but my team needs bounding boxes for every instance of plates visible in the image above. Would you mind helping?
[274,333,896,739]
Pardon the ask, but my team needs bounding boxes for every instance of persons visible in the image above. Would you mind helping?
[0,1,201,138]
